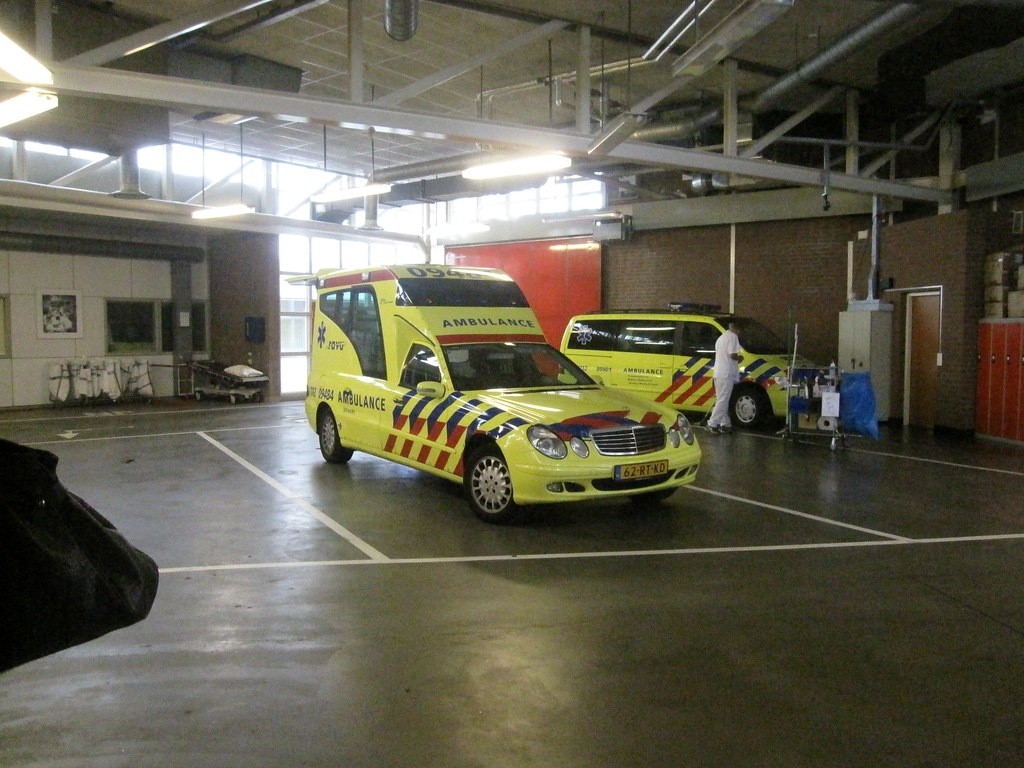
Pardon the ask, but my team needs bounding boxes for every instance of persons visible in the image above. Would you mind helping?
[508,353,557,386]
[704,321,742,436]
[454,345,504,388]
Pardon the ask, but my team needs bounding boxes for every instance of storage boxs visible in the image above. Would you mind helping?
[980,251,1024,318]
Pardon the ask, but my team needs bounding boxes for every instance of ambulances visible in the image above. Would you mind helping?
[285,263,705,524]
[560,303,826,430]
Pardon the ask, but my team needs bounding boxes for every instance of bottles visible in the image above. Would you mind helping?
[829,361,835,376]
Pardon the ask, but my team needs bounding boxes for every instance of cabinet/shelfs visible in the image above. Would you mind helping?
[837,310,893,422]
[974,317,1024,450]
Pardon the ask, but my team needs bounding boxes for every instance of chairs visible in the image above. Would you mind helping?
[476,366,505,391]
[353,313,378,331]
[668,323,697,356]
[511,351,545,389]
[699,326,716,347]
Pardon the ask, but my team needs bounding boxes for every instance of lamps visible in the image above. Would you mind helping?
[191,122,252,221]
[462,39,572,181]
[308,126,393,202]
[689,172,719,197]
[586,0,646,155]
[590,214,634,243]
[672,0,793,79]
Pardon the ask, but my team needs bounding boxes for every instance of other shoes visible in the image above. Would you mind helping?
[704,425,720,434]
[718,426,730,434]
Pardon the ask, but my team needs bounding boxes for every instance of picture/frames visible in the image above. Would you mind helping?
[34,288,84,340]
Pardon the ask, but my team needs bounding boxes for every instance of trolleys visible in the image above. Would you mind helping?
[187,360,266,405]
[781,367,864,451]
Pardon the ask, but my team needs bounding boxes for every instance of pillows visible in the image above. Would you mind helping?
[225,364,263,378]
